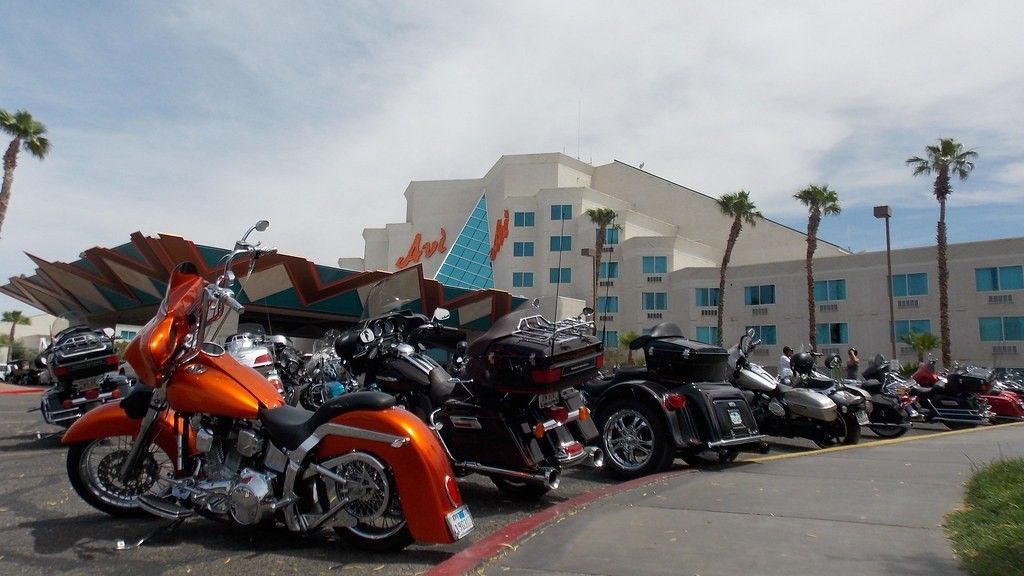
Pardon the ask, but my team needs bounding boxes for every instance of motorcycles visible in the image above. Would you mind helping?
[781,343,920,441]
[334,275,607,503]
[720,325,874,449]
[558,320,770,476]
[58,219,477,553]
[861,352,997,431]
[909,353,1024,423]
[1,310,375,460]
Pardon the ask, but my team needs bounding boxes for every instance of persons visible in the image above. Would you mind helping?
[846,349,860,381]
[779,345,794,375]
